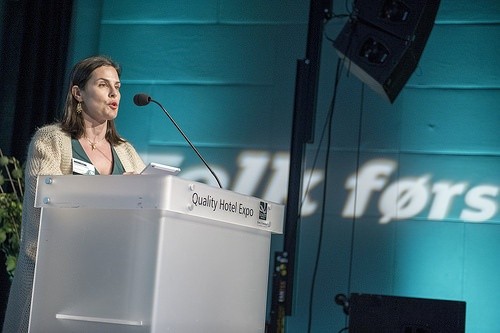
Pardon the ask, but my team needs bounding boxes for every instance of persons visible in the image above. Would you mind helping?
[3,56,146,333]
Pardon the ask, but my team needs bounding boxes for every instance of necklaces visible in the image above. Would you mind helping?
[85,136,105,150]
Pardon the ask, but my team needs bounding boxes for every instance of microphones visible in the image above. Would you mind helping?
[133,94,222,188]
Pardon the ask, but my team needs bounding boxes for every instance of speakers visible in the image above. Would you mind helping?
[349,292,466,333]
[333,17,442,104]
[351,0,441,51]
